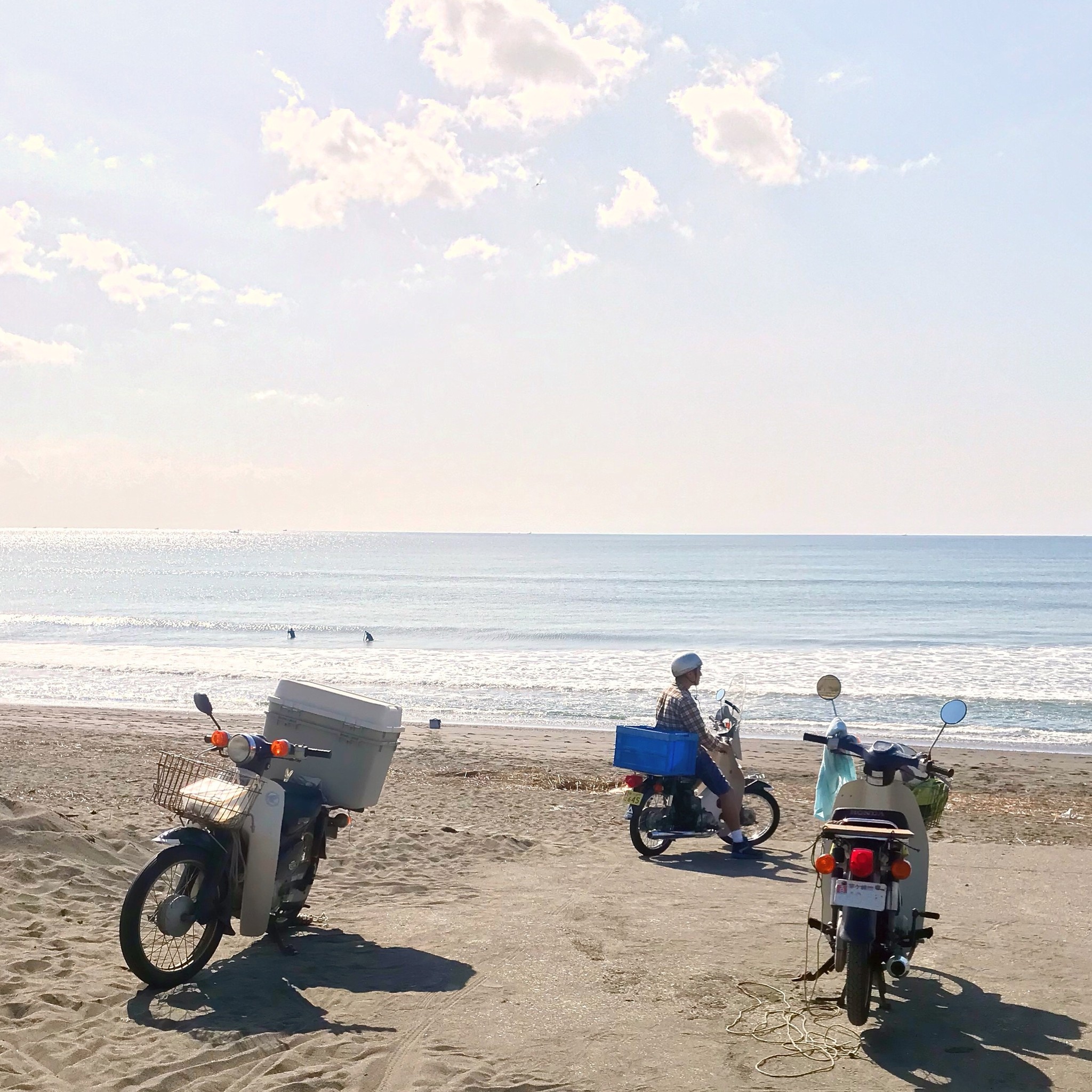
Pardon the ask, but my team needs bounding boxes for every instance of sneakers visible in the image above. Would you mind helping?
[731,838,763,859]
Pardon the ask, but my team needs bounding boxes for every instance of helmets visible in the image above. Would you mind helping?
[671,653,702,676]
[914,778,948,820]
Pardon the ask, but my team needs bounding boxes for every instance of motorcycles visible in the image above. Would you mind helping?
[623,689,780,855]
[119,693,364,990]
[791,674,967,1027]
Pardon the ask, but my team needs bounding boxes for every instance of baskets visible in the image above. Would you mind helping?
[855,764,952,832]
[151,750,267,831]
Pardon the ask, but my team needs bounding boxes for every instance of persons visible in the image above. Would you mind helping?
[654,652,766,860]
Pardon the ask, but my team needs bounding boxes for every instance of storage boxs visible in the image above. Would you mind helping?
[614,724,699,776]
[261,678,406,809]
